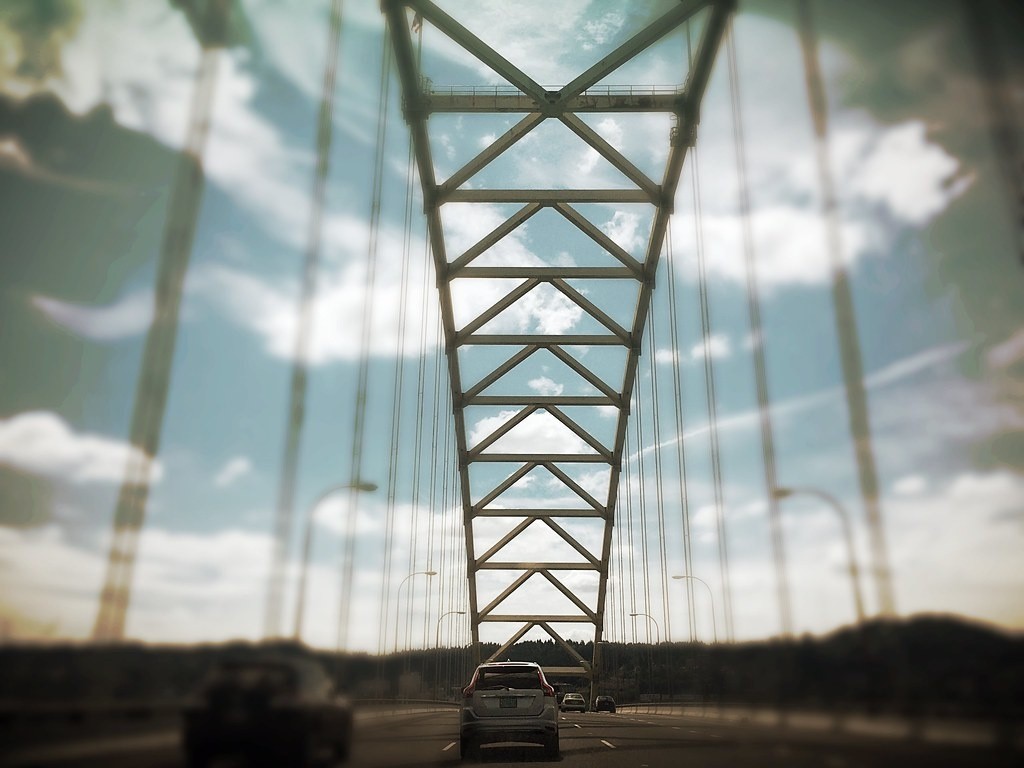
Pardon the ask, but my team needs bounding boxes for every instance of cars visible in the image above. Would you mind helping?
[559,693,586,713]
[596,695,618,714]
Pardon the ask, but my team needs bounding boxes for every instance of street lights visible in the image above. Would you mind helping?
[630,613,661,644]
[394,572,439,653]
[672,574,718,642]
[435,610,464,646]
[292,480,378,642]
[773,486,863,624]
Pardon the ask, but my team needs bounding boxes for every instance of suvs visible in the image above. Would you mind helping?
[459,660,564,761]
